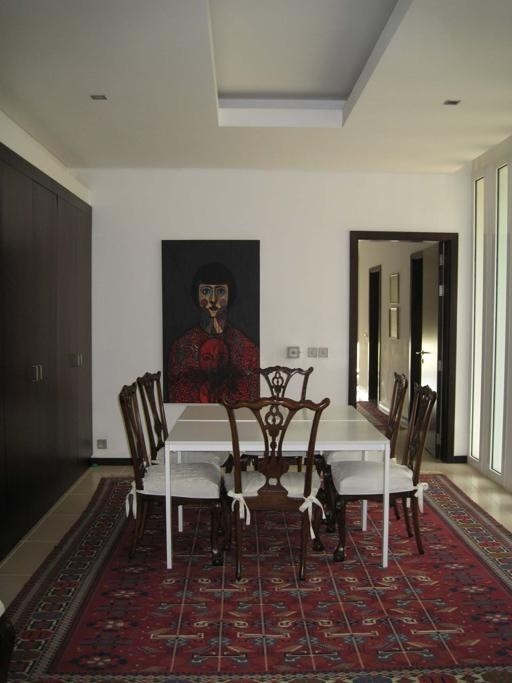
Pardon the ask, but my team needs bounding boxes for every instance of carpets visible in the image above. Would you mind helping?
[0,473,512,682]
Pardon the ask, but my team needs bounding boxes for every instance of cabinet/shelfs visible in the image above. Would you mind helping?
[0,143,93,561]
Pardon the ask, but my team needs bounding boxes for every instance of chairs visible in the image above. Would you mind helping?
[118,364,438,580]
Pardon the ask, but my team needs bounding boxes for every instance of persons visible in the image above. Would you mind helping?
[200,338,249,403]
[167,263,259,403]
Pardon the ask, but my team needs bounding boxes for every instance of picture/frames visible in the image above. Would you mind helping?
[161,239,263,404]
[388,272,400,338]
